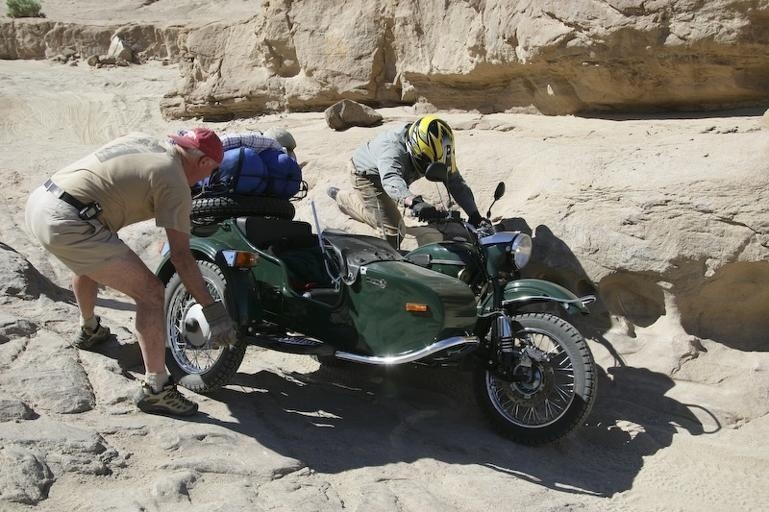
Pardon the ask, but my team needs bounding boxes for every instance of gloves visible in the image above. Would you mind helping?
[202,300,239,346]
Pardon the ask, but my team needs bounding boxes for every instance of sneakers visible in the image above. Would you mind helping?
[135,379,199,416]
[71,324,110,349]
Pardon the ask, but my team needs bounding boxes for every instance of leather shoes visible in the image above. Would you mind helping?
[44,178,89,211]
[327,187,348,216]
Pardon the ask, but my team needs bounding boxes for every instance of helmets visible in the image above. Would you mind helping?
[406,115,456,181]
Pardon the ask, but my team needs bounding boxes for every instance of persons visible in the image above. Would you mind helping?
[25,128,239,416]
[328,115,492,250]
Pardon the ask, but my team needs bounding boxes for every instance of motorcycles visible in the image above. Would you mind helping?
[154,161,598,449]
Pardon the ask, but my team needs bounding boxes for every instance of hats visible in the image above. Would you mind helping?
[168,128,223,162]
